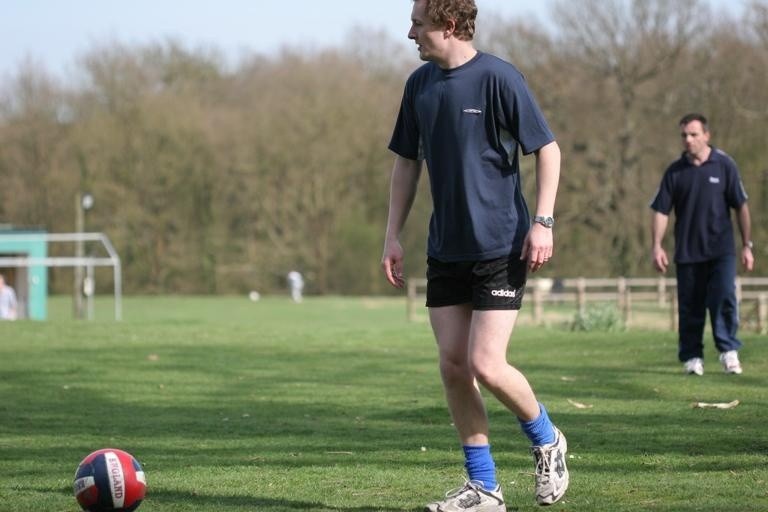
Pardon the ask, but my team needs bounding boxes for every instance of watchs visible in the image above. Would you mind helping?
[532,214,554,228]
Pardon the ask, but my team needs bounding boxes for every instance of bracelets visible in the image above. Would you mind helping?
[742,241,753,249]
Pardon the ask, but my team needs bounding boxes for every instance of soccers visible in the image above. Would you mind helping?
[73,448,147,512]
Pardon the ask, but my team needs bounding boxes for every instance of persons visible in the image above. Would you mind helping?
[284,268,305,304]
[1,272,17,320]
[650,114,757,377]
[381,1,570,511]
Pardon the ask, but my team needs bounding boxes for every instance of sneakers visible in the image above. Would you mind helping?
[530,425,569,506]
[684,357,704,376]
[719,350,742,374]
[424,476,506,512]
